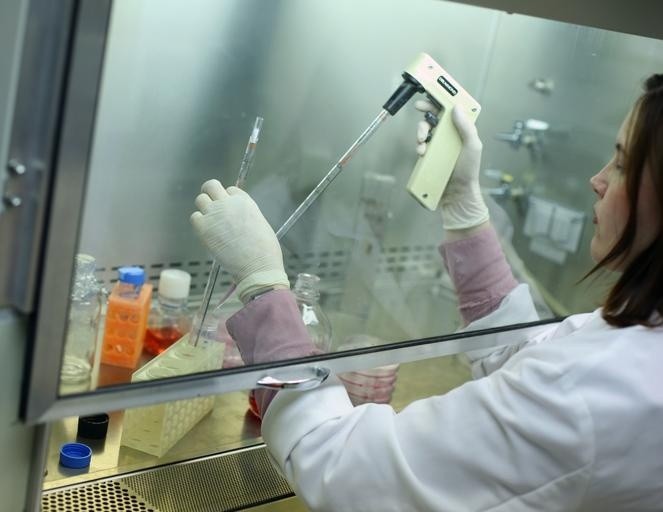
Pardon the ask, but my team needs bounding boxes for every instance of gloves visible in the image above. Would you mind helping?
[189,179,289,304]
[413,98,491,232]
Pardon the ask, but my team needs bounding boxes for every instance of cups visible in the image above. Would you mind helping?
[341,334,396,408]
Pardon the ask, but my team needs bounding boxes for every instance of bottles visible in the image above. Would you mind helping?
[247,272,333,425]
[60,252,192,386]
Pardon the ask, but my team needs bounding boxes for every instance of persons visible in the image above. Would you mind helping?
[189,73,663,512]
